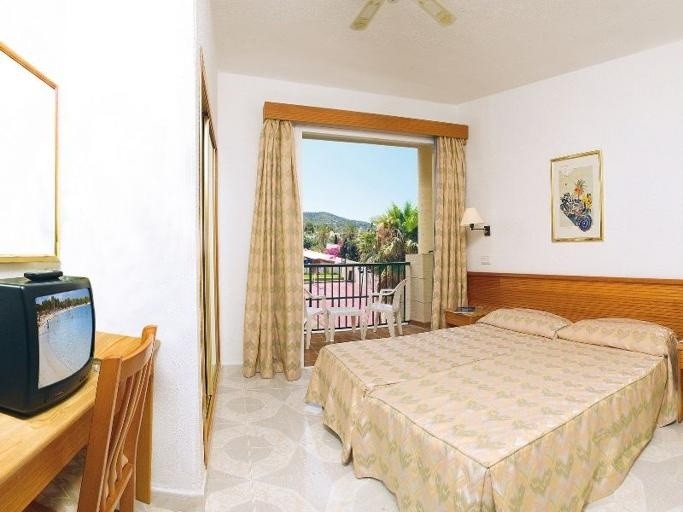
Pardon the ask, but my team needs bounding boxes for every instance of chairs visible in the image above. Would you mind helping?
[302,287,328,351]
[20,325,161,508]
[363,279,406,339]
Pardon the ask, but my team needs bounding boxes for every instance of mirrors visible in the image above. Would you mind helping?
[0,41,65,266]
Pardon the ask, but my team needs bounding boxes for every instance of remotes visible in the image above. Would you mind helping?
[23,270,63,281]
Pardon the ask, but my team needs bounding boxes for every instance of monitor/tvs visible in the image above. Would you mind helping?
[0,275,95,417]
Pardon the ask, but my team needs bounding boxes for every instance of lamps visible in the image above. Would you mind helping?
[458,207,491,236]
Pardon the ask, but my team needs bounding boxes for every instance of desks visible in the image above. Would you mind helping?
[1,333,154,510]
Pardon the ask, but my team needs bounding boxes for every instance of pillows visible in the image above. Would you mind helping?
[477,306,572,339]
[555,317,678,357]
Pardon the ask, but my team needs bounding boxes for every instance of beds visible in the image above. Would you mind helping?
[314,271,683,512]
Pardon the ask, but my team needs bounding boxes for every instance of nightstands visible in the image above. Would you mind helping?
[444,306,489,328]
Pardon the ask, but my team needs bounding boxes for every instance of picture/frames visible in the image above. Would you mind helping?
[549,149,604,243]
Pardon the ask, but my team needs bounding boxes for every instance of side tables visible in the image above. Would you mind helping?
[329,307,365,342]
